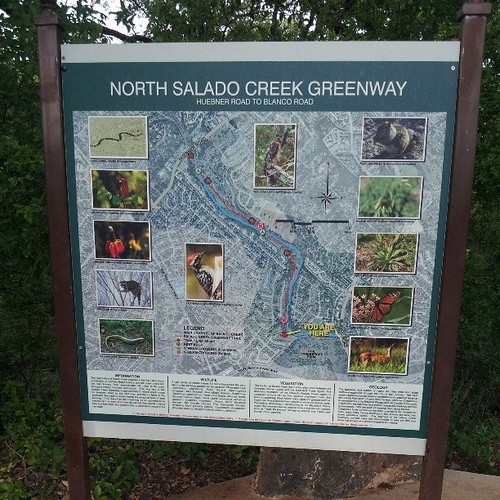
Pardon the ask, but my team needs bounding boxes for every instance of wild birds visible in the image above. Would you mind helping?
[187,249,222,299]
[118,178,128,196]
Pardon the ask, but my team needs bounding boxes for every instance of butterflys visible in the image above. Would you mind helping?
[369,292,401,320]
